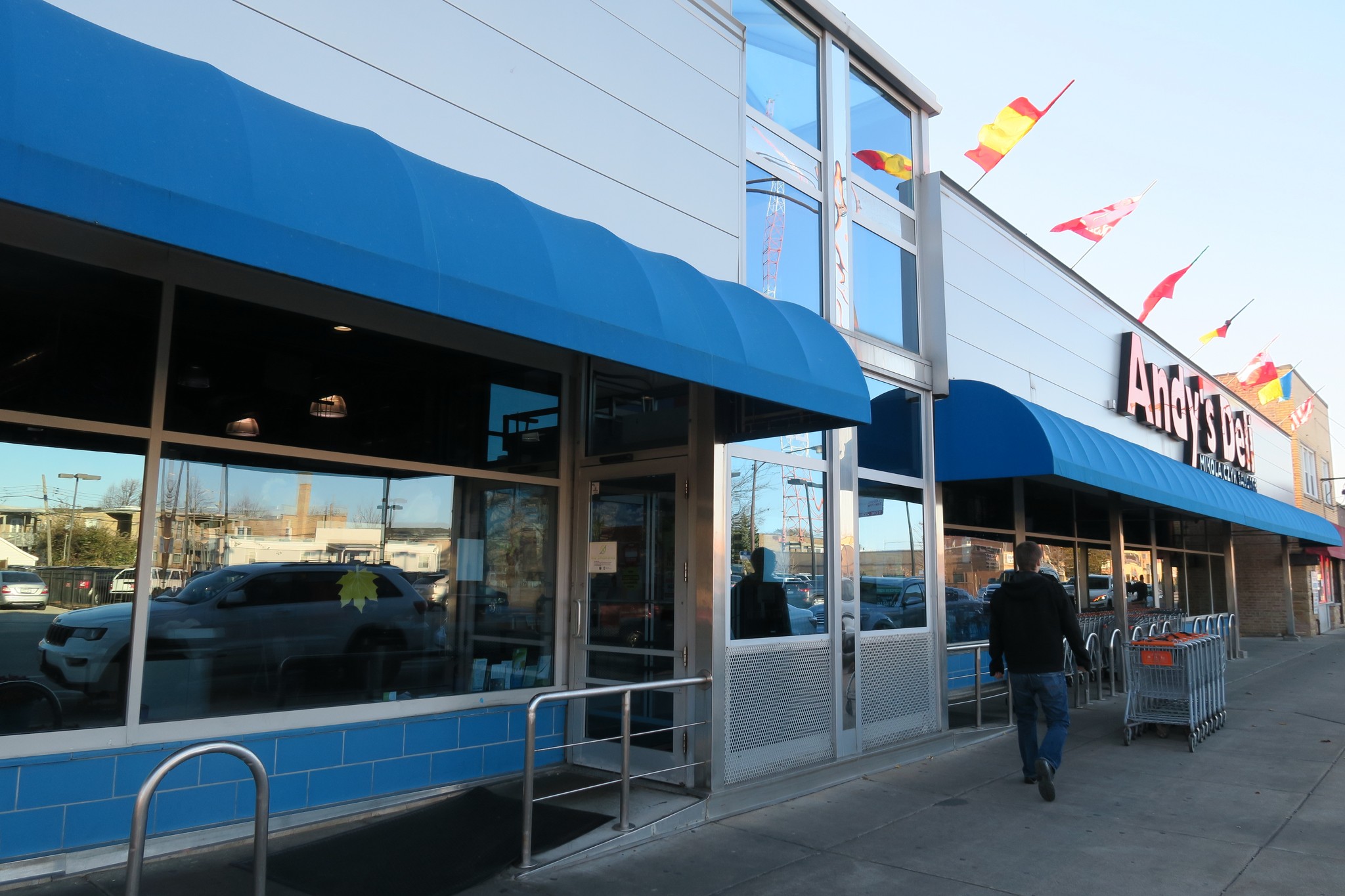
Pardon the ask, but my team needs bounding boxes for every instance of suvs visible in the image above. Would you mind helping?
[807,571,926,634]
[1065,574,1115,612]
[63,572,114,605]
[38,560,445,714]
[109,566,190,604]
[412,574,509,619]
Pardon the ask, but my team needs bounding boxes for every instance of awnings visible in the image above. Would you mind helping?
[934,380,1343,546]
[0,0,871,443]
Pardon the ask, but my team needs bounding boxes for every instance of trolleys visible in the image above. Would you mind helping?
[1063,608,1226,754]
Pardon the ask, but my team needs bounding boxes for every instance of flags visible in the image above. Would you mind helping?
[1237,348,1278,390]
[1289,398,1313,431]
[1197,320,1231,346]
[1049,195,1144,242]
[1218,336,1219,337]
[1138,263,1193,324]
[964,96,1044,172]
[1257,371,1291,405]
[852,150,913,180]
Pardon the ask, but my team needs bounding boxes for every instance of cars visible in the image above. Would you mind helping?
[1146,585,1163,608]
[730,572,823,640]
[0,570,49,610]
[945,586,985,644]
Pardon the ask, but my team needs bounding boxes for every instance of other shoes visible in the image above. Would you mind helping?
[1034,758,1055,802]
[1024,776,1038,784]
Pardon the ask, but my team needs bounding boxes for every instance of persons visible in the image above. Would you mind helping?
[1127,575,1147,603]
[731,546,792,641]
[988,540,1094,802]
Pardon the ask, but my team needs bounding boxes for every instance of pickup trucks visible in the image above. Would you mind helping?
[978,567,1076,615]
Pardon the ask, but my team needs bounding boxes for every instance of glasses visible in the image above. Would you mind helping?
[773,561,777,566]
[1040,558,1043,563]
